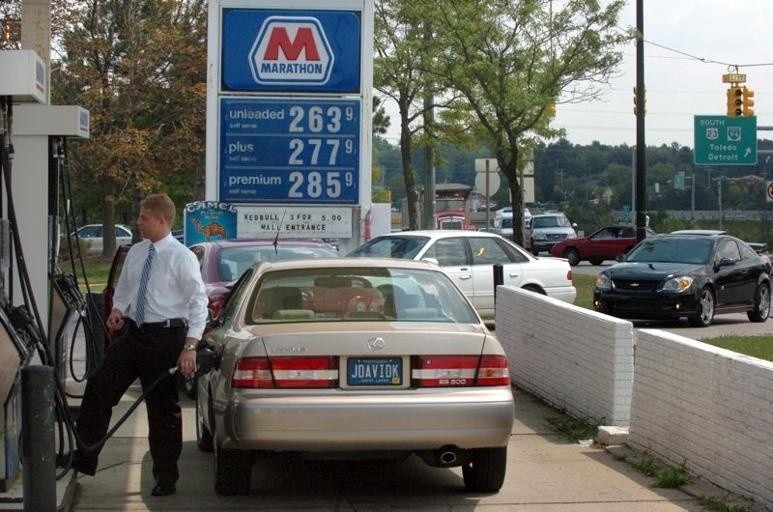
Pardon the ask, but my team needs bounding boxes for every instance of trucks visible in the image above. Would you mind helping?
[492,206,533,228]
[395,181,483,231]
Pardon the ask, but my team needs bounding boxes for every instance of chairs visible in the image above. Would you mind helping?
[260,287,303,320]
[385,293,420,318]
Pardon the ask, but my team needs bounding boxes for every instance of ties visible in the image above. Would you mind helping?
[136,244,155,328]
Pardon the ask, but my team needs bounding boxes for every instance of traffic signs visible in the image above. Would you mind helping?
[692,114,759,166]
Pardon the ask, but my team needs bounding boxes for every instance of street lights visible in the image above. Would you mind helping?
[664,174,696,220]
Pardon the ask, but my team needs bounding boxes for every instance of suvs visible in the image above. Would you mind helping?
[524,210,577,257]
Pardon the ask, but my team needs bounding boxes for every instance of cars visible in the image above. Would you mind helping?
[338,228,581,329]
[548,222,657,269]
[476,224,512,243]
[590,227,772,328]
[181,208,350,405]
[66,222,133,255]
[177,253,523,499]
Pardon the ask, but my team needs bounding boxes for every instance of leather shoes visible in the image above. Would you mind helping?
[152,484,176,496]
[56,452,97,476]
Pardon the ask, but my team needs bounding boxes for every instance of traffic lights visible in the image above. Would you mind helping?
[726,86,742,116]
[631,84,648,117]
[741,85,755,117]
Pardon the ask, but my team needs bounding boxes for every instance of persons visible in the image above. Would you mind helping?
[57,192,212,496]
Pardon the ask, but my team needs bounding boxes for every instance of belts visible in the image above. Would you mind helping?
[144,318,187,328]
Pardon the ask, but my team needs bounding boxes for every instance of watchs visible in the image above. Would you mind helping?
[183,343,198,351]
